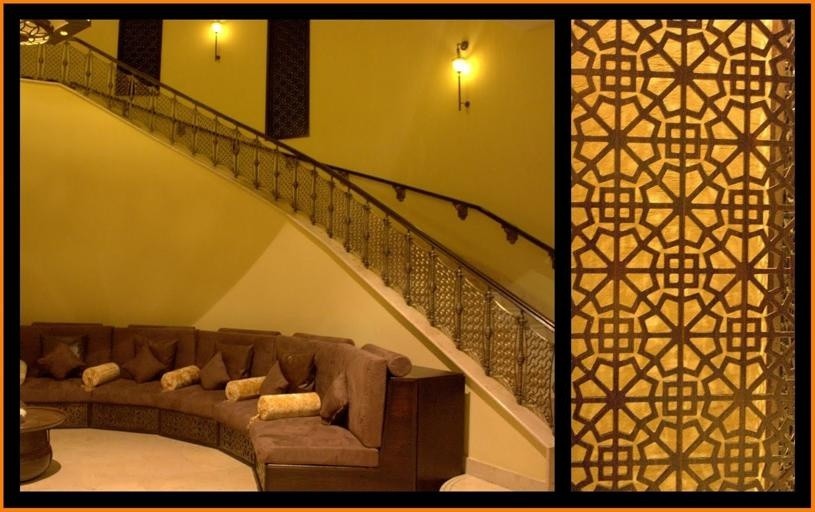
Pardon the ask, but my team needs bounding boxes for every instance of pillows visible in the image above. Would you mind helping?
[259,360,288,397]
[82,362,120,386]
[131,334,179,371]
[321,369,350,424]
[225,377,266,401]
[37,342,83,380]
[54,333,90,359]
[256,391,320,420]
[281,352,317,392]
[197,352,229,390]
[121,340,166,384]
[214,341,257,379]
[19,359,28,386]
[161,365,199,390]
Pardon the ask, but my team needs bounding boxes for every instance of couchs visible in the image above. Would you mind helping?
[18,327,466,490]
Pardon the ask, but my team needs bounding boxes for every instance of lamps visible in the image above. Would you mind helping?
[210,20,225,61]
[451,41,470,113]
[19,19,53,47]
